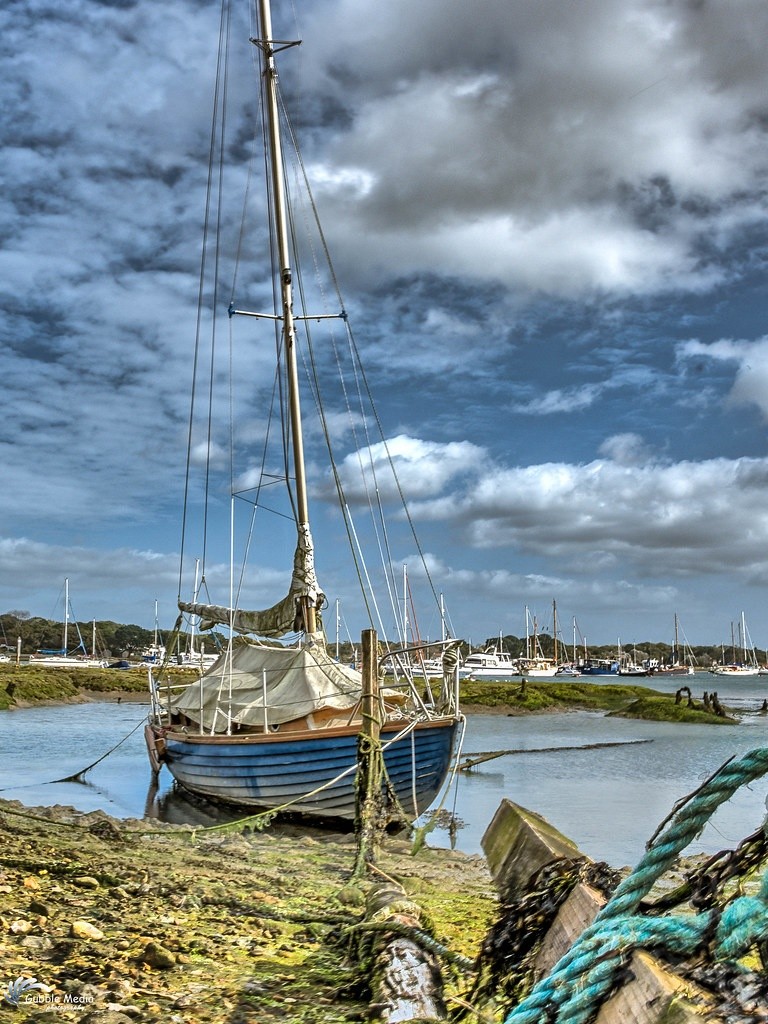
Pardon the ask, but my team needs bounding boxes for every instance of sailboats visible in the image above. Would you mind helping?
[706,610,768,677]
[324,562,694,680]
[27,576,222,674]
[144,1,465,841]
[0,615,13,664]
